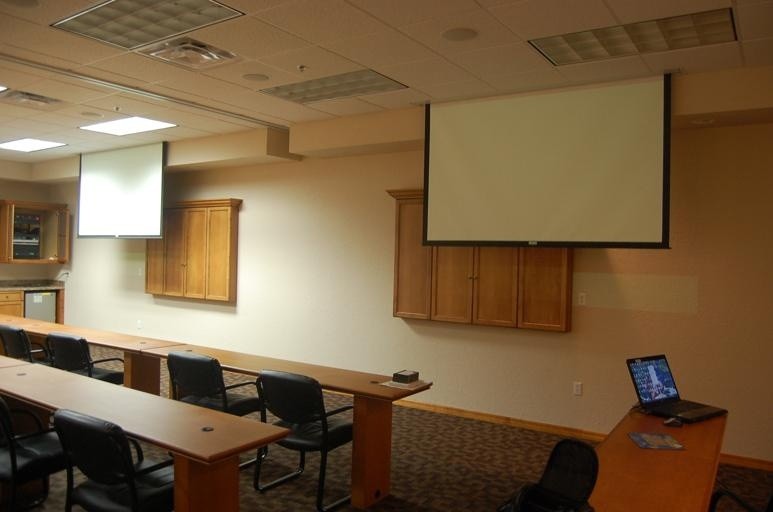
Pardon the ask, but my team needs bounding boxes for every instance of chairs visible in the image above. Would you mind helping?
[0,315,355,512]
[497,437,599,512]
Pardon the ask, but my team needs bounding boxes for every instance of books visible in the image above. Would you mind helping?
[627,431,683,449]
[390,380,424,388]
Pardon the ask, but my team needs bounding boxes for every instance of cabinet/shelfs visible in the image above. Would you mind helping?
[0,198,70,264]
[385,188,574,334]
[144,198,244,303]
[0,290,24,317]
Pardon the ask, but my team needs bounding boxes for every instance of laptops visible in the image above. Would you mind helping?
[626,354,729,422]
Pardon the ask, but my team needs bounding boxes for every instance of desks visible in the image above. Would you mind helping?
[141,341,434,512]
[586,399,728,512]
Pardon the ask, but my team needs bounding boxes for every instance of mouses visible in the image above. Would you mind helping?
[664,417,682,426]
